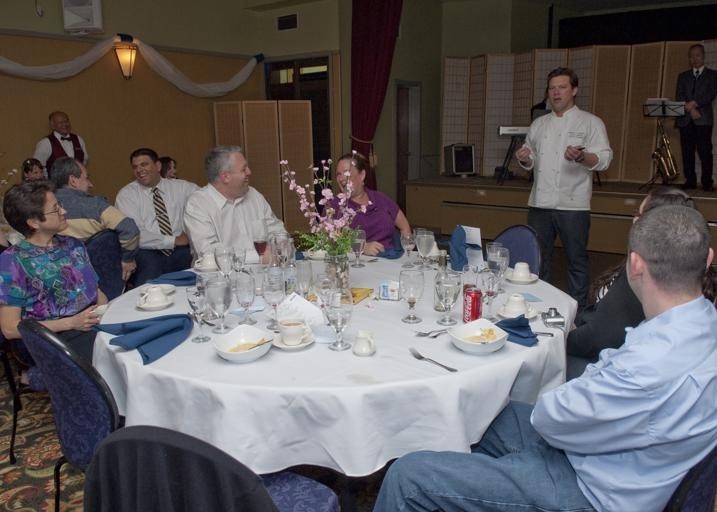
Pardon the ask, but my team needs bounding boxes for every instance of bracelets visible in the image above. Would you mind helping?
[446,316,507,356]
[575,152,585,163]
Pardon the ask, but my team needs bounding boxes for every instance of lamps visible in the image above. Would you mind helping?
[84,228,128,302]
[393,226,403,247]
[17,316,123,512]
[665,444,717,512]
[84,425,341,512]
[491,223,548,279]
[0,332,48,464]
[174,244,193,269]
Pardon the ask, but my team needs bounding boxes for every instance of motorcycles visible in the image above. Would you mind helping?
[638,117,685,191]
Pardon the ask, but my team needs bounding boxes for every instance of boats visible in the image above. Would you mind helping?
[444,142,475,178]
[62,0,103,33]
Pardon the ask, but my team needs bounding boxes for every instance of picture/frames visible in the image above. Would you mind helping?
[91,256,580,511]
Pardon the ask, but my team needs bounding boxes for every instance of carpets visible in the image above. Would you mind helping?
[149,187,175,257]
[694,70,700,80]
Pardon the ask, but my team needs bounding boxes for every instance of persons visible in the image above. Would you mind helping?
[1,182,110,360]
[22,158,45,182]
[673,44,716,193]
[320,153,414,256]
[513,67,615,310]
[33,110,88,180]
[182,146,290,269]
[51,157,140,281]
[115,147,202,285]
[370,204,717,512]
[568,185,699,381]
[159,157,179,179]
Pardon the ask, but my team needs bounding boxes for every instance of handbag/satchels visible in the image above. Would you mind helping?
[575,152,585,163]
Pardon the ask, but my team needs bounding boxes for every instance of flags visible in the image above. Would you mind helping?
[324,254,351,298]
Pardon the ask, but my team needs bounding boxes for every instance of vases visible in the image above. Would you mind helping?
[277,150,370,286]
[0,151,22,194]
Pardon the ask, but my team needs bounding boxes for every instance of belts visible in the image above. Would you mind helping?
[398,226,509,326]
[321,289,351,350]
[185,231,325,363]
[349,231,368,270]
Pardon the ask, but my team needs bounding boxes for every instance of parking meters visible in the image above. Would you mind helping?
[652,119,681,180]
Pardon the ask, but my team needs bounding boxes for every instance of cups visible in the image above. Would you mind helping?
[31,199,63,219]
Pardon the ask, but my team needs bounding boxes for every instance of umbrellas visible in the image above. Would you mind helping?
[678,181,714,193]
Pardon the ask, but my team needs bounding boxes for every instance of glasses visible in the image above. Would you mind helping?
[112,33,137,80]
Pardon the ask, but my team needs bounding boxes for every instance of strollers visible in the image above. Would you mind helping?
[60,136,72,142]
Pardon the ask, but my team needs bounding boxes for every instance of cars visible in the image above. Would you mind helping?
[513,262,530,280]
[142,287,166,304]
[504,294,528,316]
[378,279,404,301]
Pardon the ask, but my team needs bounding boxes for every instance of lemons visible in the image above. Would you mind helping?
[351,330,376,357]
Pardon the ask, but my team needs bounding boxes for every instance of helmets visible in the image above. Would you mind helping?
[502,272,538,285]
[135,301,174,311]
[496,305,538,322]
[138,284,176,294]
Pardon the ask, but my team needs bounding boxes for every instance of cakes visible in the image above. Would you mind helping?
[462,288,482,323]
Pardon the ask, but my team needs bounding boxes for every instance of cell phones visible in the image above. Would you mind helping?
[541,308,565,327]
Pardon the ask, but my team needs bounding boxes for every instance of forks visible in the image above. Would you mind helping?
[410,345,455,374]
